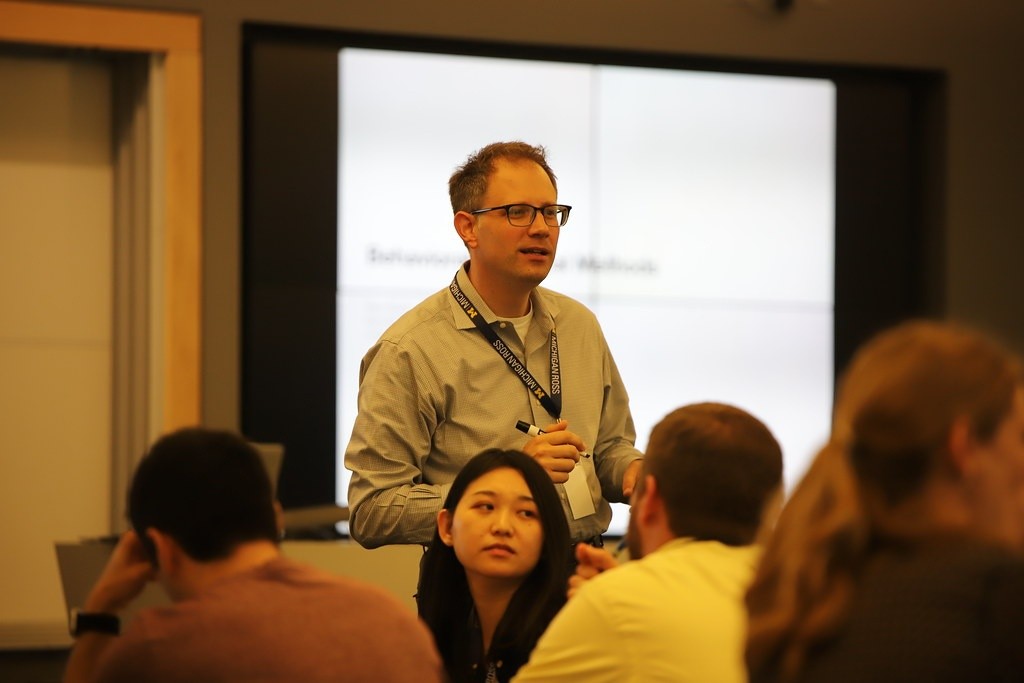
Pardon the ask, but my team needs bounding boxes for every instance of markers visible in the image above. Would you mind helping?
[515,419,591,459]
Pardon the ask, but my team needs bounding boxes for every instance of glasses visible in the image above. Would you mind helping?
[470,204,573,227]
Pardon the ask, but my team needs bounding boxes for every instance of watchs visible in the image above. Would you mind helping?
[69,610,120,637]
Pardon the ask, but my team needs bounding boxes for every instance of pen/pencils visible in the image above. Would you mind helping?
[608,534,629,559]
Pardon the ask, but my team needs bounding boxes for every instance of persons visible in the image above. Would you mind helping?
[510,401,785,682]
[417,448,576,682]
[344,142,653,622]
[64,426,443,682]
[745,318,1024,683]
